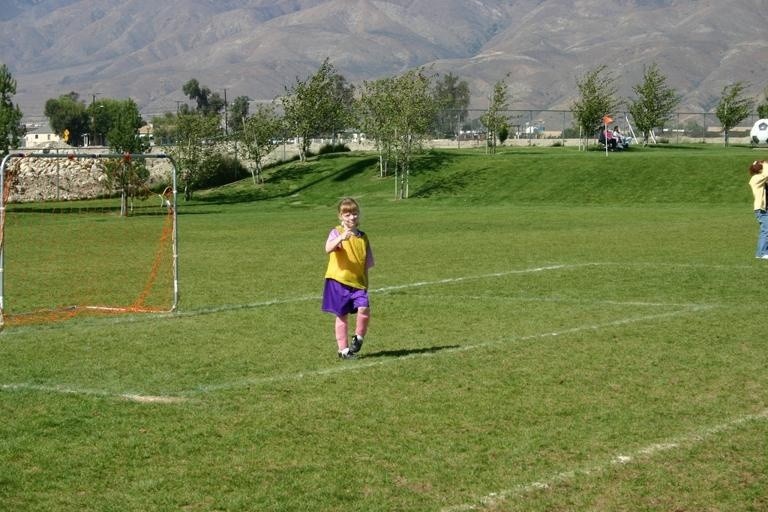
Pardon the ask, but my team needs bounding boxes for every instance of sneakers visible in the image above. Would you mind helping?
[349,335,363,352]
[338,348,360,361]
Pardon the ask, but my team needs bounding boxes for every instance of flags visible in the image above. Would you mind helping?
[603,115,613,124]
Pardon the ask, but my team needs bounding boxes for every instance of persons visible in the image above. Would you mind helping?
[320,198,374,362]
[613,126,632,148]
[599,125,615,152]
[746,159,768,258]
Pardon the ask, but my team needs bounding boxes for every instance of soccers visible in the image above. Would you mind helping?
[751,119,768,144]
